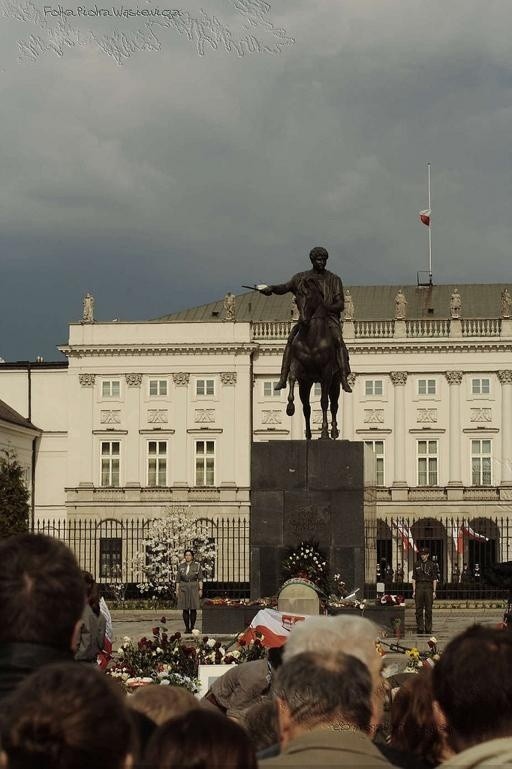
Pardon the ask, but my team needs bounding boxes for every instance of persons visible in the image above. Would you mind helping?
[174,550,204,633]
[262,247,352,394]
[393,289,408,319]
[412,548,439,634]
[452,563,460,584]
[499,288,512,319]
[449,288,462,318]
[473,564,481,582]
[343,289,354,320]
[384,563,394,582]
[222,290,236,321]
[461,563,470,578]
[0,615,512,769]
[81,291,95,324]
[0,533,85,688]
[72,568,108,664]
[394,563,405,582]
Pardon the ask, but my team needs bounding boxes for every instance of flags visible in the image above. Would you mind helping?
[460,520,488,543]
[401,519,418,553]
[390,520,411,553]
[449,519,465,554]
[419,209,432,225]
[237,608,309,647]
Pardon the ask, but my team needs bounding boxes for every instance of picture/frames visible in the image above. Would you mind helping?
[198,664,238,700]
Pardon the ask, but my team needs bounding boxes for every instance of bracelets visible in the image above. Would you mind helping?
[199,589,202,590]
[432,589,437,592]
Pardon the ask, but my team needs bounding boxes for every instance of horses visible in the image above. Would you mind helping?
[287,274,339,440]
